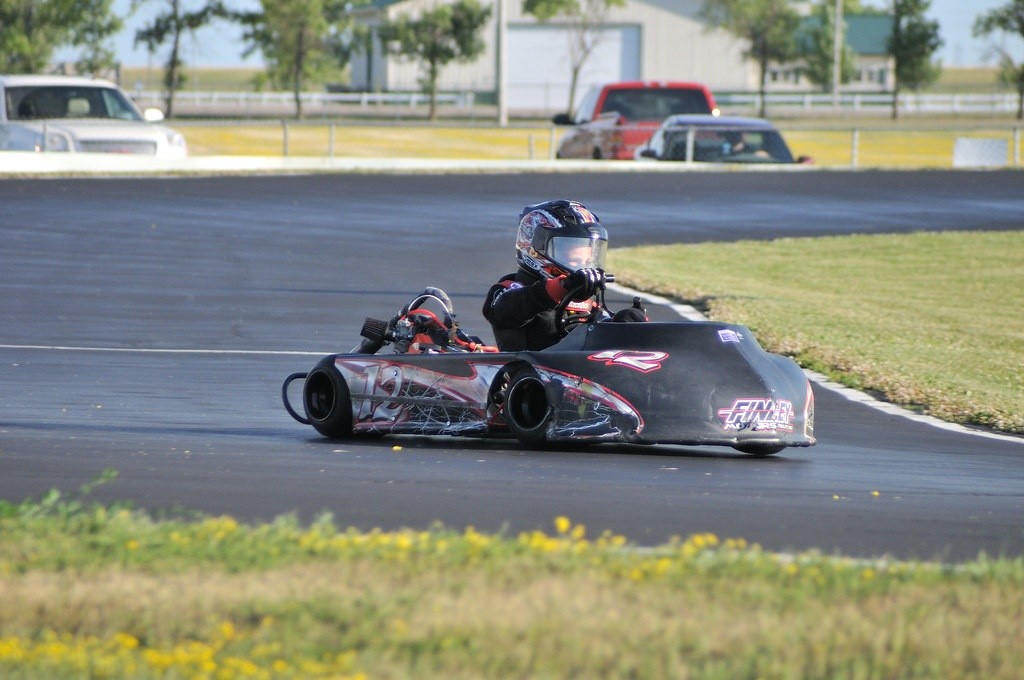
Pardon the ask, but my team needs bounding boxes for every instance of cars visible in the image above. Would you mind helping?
[636,114,812,164]
[0,74,187,157]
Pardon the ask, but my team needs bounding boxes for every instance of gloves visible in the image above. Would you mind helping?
[564,267,606,300]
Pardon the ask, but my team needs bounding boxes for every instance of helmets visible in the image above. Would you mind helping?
[516,200,609,280]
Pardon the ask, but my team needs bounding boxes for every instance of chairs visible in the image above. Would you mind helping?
[10,95,44,119]
[672,96,701,113]
[64,97,91,117]
[674,140,707,160]
[612,103,637,122]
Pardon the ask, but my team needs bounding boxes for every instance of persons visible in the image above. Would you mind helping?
[481,199,649,351]
[725,131,768,158]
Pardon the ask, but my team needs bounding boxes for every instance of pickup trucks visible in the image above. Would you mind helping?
[553,82,720,160]
